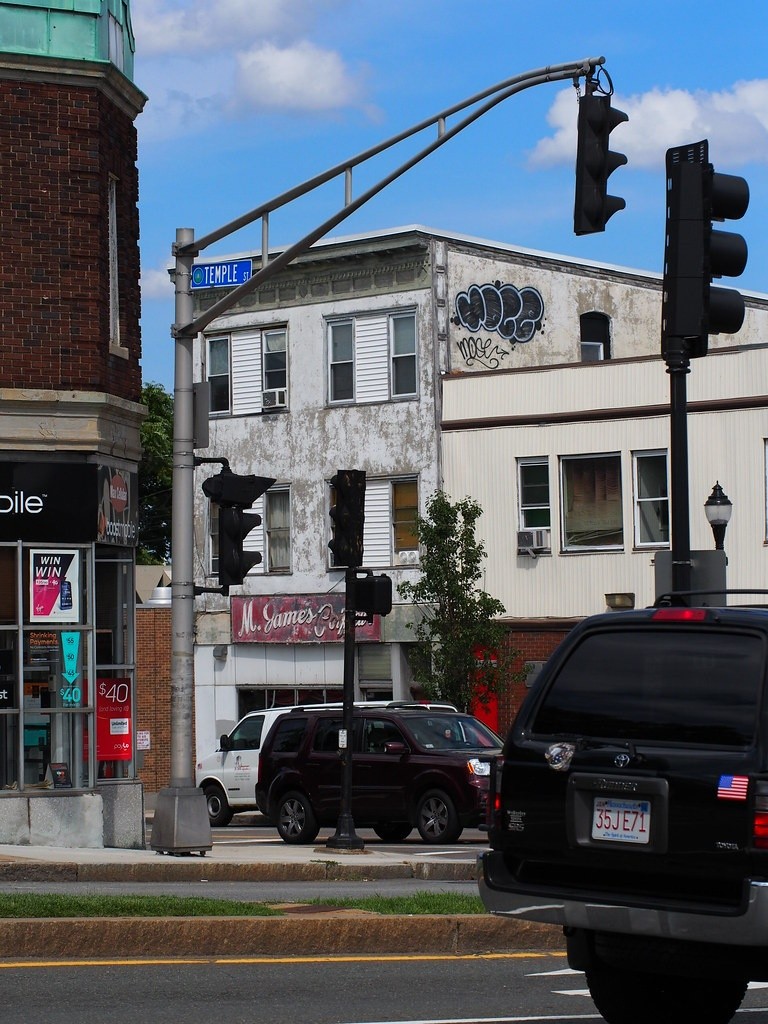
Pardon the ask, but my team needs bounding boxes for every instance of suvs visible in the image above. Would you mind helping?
[477,588,768,1024]
[254,705,506,844]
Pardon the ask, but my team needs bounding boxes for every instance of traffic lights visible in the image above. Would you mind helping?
[202,473,276,585]
[664,139,748,371]
[573,95,629,236]
[328,469,366,567]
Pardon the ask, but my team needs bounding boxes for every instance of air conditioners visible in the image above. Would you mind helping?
[261,388,288,408]
[517,530,548,550]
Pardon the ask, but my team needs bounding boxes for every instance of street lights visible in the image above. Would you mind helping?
[702,479,732,561]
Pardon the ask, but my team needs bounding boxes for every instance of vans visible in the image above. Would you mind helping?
[195,702,456,829]
